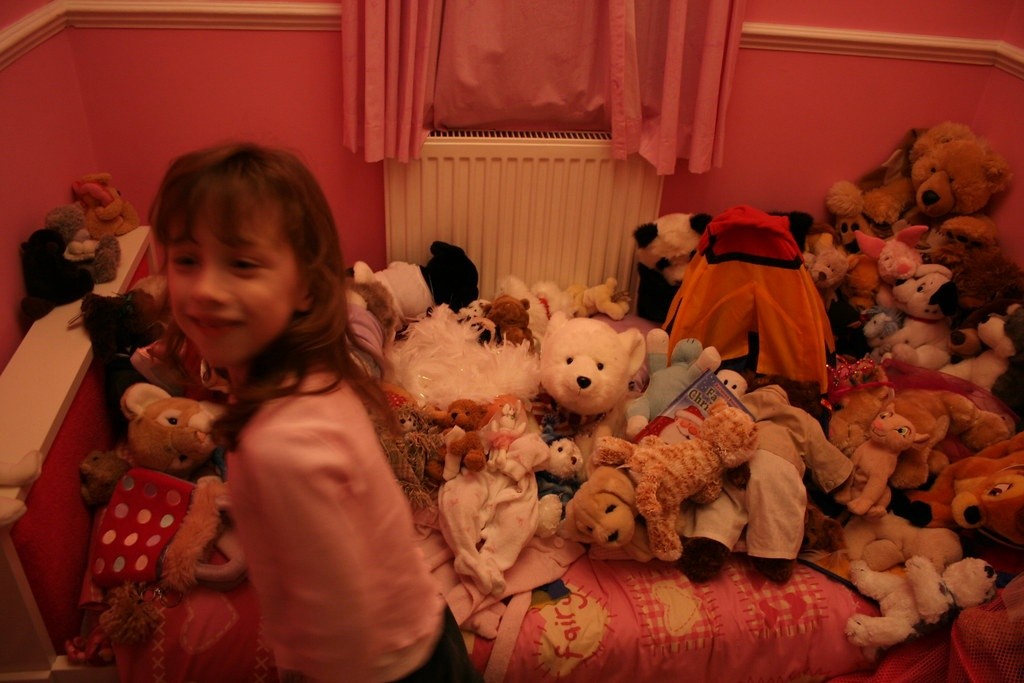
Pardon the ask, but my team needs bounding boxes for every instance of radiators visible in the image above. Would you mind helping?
[383,128,664,318]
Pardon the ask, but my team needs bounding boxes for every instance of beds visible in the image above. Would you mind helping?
[0,225,887,683]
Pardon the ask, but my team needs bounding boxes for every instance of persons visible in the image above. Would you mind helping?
[146,142,481,683]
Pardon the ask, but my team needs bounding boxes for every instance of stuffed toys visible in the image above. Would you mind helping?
[19,121,1024,683]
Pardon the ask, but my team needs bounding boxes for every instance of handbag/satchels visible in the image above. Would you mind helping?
[90,468,248,590]
